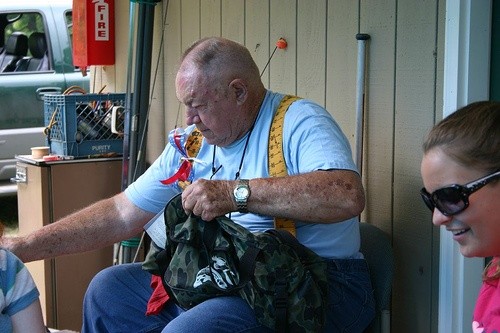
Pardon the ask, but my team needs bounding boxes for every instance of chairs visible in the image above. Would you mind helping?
[359,221,394,333]
[14,31,50,73]
[0,31,28,73]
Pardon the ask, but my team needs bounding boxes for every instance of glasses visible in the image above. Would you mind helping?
[420,171,500,217]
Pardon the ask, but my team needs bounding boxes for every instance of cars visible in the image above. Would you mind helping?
[0,1,90,197]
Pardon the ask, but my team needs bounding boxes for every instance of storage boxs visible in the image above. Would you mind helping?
[42,94,136,158]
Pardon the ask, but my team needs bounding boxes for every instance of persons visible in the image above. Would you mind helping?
[0,37,376,333]
[0,245,49,333]
[420,101,500,333]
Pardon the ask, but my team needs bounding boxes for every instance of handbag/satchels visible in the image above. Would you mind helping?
[143,190,337,333]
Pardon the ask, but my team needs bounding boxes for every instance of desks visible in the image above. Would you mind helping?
[15,153,133,333]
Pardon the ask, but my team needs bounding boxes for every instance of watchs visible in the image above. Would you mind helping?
[232,179,251,214]
